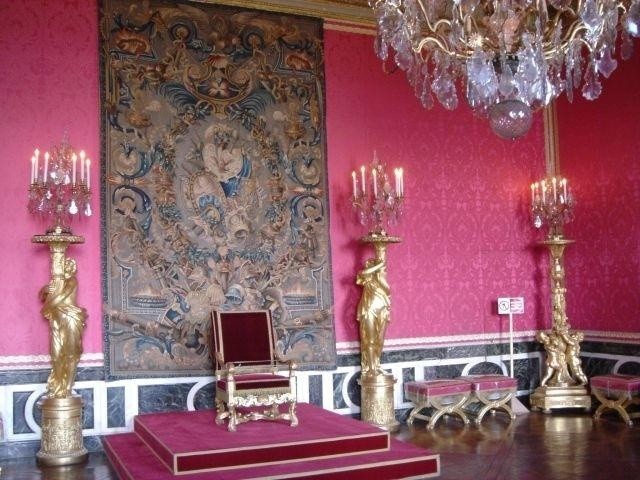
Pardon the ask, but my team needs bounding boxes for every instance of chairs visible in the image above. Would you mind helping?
[209,308,300,431]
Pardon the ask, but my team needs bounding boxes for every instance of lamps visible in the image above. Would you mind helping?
[346,146,406,236]
[527,174,575,239]
[26,131,97,236]
[365,0,640,140]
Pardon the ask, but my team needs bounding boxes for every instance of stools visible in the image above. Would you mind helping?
[587,374,640,428]
[402,372,518,432]
